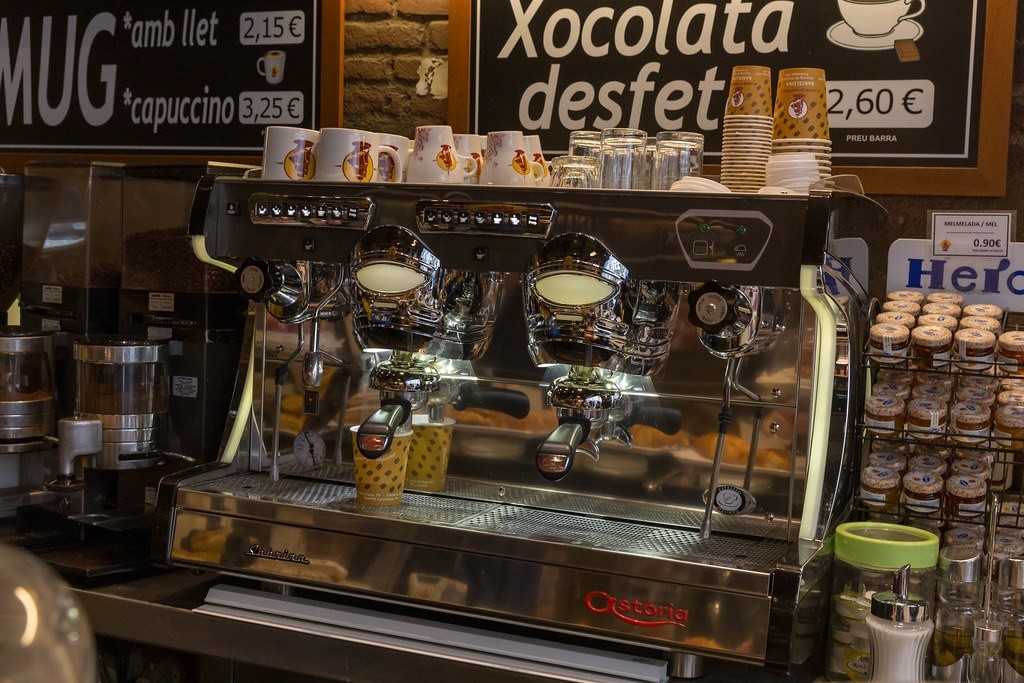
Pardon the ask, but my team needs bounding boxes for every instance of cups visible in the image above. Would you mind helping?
[404,415,456,491]
[350,424,405,509]
[260,125,551,189]
[938,544,1024,683]
[720,65,837,196]
[551,127,705,192]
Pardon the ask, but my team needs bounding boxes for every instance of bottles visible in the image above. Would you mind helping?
[863,564,933,683]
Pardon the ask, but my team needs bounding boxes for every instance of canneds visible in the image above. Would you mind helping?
[858,290,1024,580]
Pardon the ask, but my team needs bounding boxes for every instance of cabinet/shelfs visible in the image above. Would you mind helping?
[853,297,1024,534]
